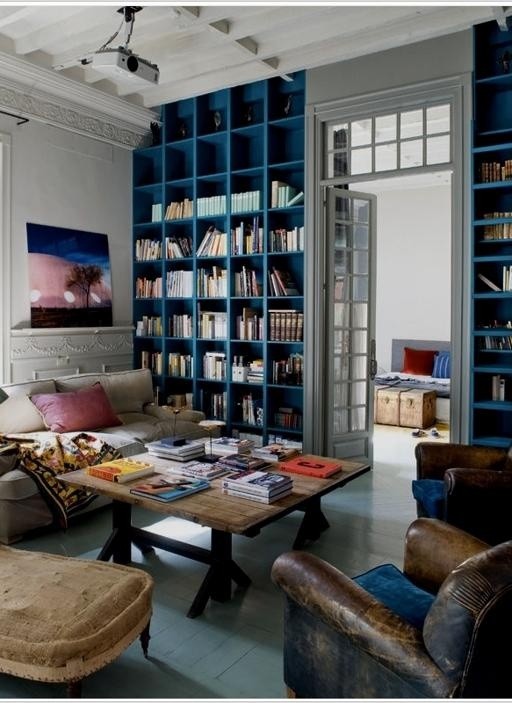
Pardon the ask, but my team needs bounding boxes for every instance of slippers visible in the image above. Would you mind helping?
[411,430,427,437]
[429,428,439,436]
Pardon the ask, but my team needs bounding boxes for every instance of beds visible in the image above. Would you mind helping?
[375,338,451,426]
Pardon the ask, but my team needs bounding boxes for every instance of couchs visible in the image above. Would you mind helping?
[269,517,512,699]
[0,367,221,546]
[410,442,512,525]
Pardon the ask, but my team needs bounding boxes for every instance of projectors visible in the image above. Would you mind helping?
[91,47,159,90]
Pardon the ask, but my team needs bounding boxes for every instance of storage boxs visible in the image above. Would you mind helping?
[373,386,437,429]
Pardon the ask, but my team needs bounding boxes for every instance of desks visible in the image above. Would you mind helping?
[53,436,372,619]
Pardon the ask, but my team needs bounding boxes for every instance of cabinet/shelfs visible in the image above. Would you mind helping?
[131,68,310,455]
[468,14,511,451]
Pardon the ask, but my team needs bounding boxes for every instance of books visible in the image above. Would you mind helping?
[477,158,512,185]
[254,442,299,464]
[230,216,263,256]
[483,316,512,350]
[132,238,162,262]
[234,265,263,297]
[272,405,305,431]
[480,212,512,241]
[167,312,192,339]
[221,469,294,505]
[166,387,194,409]
[196,193,225,216]
[169,460,230,481]
[196,302,228,339]
[134,472,210,501]
[134,314,163,337]
[236,392,260,427]
[246,360,264,384]
[266,226,304,253]
[152,382,161,408]
[205,436,255,454]
[202,351,227,380]
[144,435,206,462]
[195,225,227,257]
[166,270,193,299]
[269,266,301,297]
[271,180,304,208]
[163,198,194,220]
[134,276,162,300]
[199,389,228,421]
[140,350,163,377]
[165,234,193,259]
[150,203,162,222]
[214,453,274,473]
[167,352,194,379]
[271,354,305,385]
[279,455,343,477]
[86,458,155,484]
[196,266,228,299]
[491,373,511,402]
[231,190,263,213]
[235,305,264,340]
[267,309,305,342]
[477,265,512,291]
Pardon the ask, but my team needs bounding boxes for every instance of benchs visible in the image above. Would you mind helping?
[0,544,154,698]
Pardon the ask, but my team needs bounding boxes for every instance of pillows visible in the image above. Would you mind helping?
[431,354,451,378]
[438,351,451,355]
[25,380,125,434]
[400,347,438,376]
